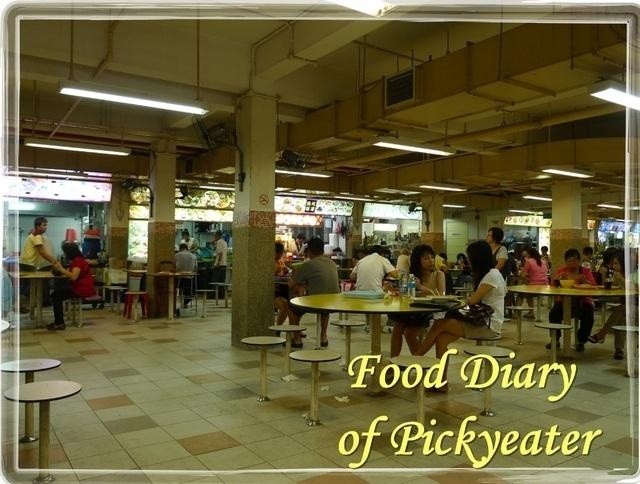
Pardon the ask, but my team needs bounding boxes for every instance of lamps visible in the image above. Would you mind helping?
[24,82,132,156]
[59,19,212,115]
[372,118,456,157]
[589,80,640,111]
[418,182,468,192]
[541,111,595,178]
[275,148,334,177]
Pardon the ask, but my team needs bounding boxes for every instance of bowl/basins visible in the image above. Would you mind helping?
[414,297,448,304]
[52,271,62,276]
[559,280,575,289]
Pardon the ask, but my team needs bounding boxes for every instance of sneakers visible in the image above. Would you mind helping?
[47,322,66,330]
[546,342,560,348]
[321,340,328,347]
[292,334,306,348]
[614,351,623,359]
[430,386,448,393]
[589,335,604,343]
[523,314,535,319]
[577,342,584,352]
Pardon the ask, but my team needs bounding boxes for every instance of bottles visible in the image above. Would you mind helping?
[407,274,416,299]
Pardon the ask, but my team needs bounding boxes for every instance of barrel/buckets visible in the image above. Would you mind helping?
[82,235,102,259]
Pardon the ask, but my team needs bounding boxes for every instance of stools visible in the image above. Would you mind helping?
[0,283,638,484]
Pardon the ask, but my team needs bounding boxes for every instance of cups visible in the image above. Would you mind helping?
[605,282,613,289]
[554,279,560,288]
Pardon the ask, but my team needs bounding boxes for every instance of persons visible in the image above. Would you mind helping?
[175,228,638,392]
[13,218,57,313]
[29,229,51,307]
[47,243,96,330]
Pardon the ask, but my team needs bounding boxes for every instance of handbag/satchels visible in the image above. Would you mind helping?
[446,302,493,327]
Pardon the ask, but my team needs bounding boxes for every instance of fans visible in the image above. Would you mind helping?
[191,116,244,192]
[274,148,308,173]
[406,202,429,232]
[175,174,198,206]
[109,170,153,218]
